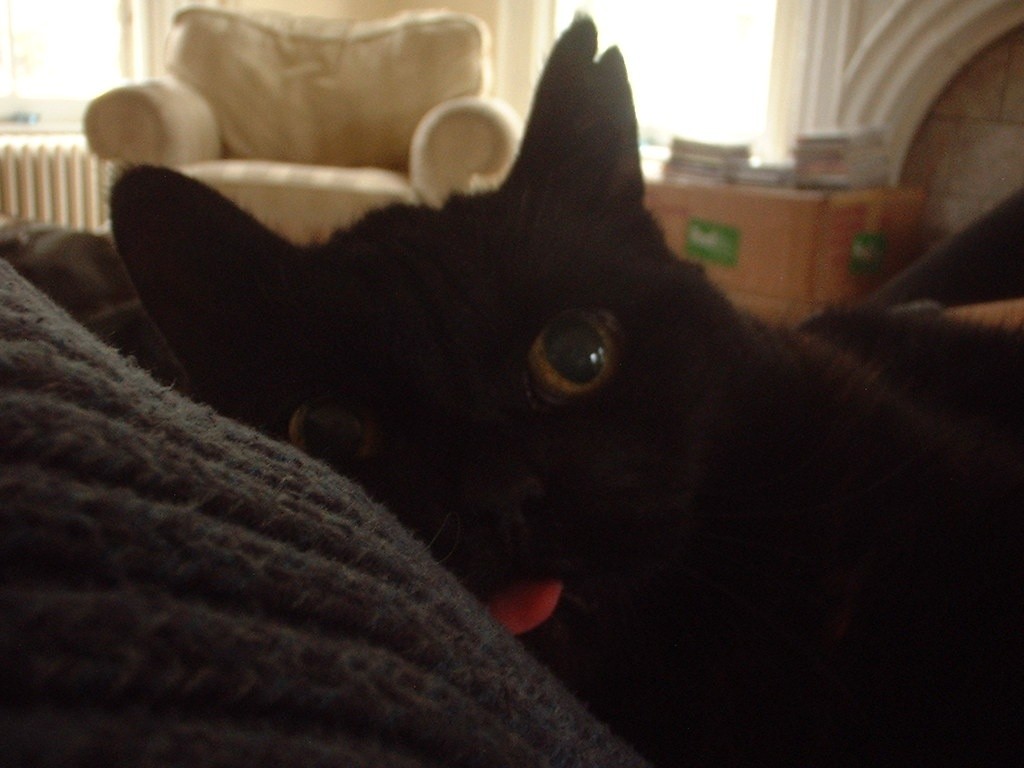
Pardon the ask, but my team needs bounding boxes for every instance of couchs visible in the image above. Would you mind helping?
[81,4,522,241]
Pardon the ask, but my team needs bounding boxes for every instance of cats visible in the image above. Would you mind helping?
[109,18,1024,768]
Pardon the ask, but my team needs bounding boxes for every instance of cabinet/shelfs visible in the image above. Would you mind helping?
[645,178,928,321]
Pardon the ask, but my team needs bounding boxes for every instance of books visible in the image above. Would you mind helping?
[662,128,892,190]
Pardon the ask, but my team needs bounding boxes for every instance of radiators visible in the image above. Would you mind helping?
[0,143,113,232]
